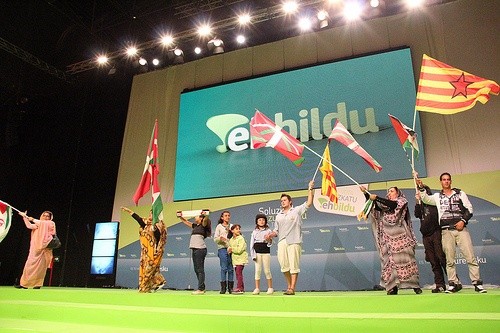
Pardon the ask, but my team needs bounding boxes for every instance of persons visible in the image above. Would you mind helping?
[417,173,488,294]
[227,225,249,292]
[120,206,167,292]
[214,211,234,294]
[250,214,274,295]
[15,210,56,289]
[179,214,211,295]
[270,180,314,295]
[415,184,461,293]
[360,185,422,294]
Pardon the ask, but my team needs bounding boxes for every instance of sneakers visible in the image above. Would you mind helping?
[445,286,458,293]
[475,285,487,293]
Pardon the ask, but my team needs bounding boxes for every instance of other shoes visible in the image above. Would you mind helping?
[233,288,244,293]
[33,286,40,289]
[154,281,164,291]
[267,288,274,295]
[191,289,206,295]
[283,288,290,295]
[252,288,260,294]
[286,288,295,295]
[15,285,28,289]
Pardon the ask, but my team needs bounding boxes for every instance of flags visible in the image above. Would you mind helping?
[416,54,500,115]
[320,145,338,203]
[389,114,420,158]
[134,122,163,225]
[249,111,305,167]
[330,120,383,173]
[0,202,13,243]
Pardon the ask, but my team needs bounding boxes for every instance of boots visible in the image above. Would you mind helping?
[220,281,226,294]
[227,280,234,294]
